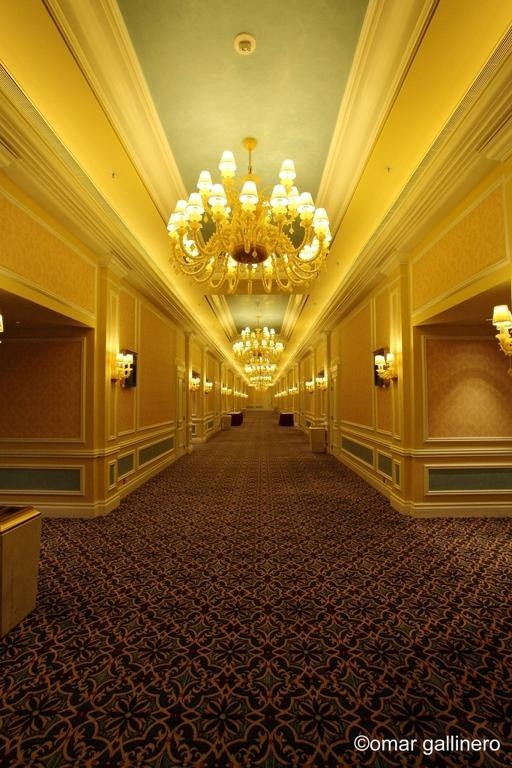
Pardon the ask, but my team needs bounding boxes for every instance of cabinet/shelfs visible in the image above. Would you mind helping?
[221,415,231,430]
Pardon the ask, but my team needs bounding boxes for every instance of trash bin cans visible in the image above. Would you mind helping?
[221,415,232,430]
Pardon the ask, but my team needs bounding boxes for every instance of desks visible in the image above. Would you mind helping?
[279,412,294,426]
[228,411,243,426]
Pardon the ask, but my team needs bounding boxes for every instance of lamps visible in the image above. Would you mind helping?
[229,301,285,386]
[374,353,397,383]
[166,31,333,299]
[492,303,512,357]
[315,377,326,391]
[111,354,132,383]
[191,378,200,392]
